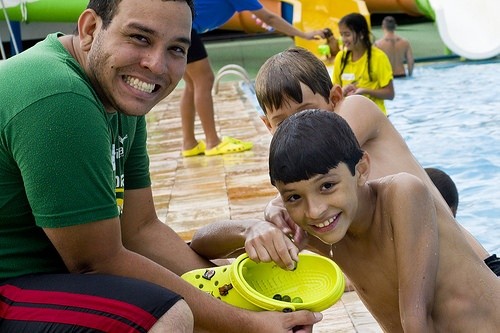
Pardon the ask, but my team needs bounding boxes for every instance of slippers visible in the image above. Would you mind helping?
[203,135,253,157]
[180,139,205,157]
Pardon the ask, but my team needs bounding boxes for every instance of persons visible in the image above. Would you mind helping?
[179,0,500,333]
[0,0,324,333]
